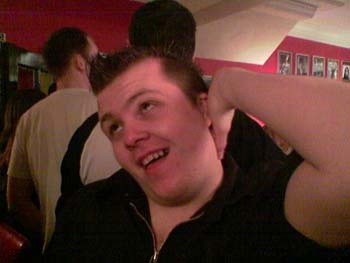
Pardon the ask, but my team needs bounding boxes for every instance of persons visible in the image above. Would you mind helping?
[37,38,350,263]
[6,25,98,263]
[280,52,350,81]
[0,88,57,248]
[37,0,294,231]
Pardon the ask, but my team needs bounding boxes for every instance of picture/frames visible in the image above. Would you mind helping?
[327,58,339,80]
[277,50,293,77]
[295,53,309,77]
[341,61,350,81]
[312,56,325,78]
